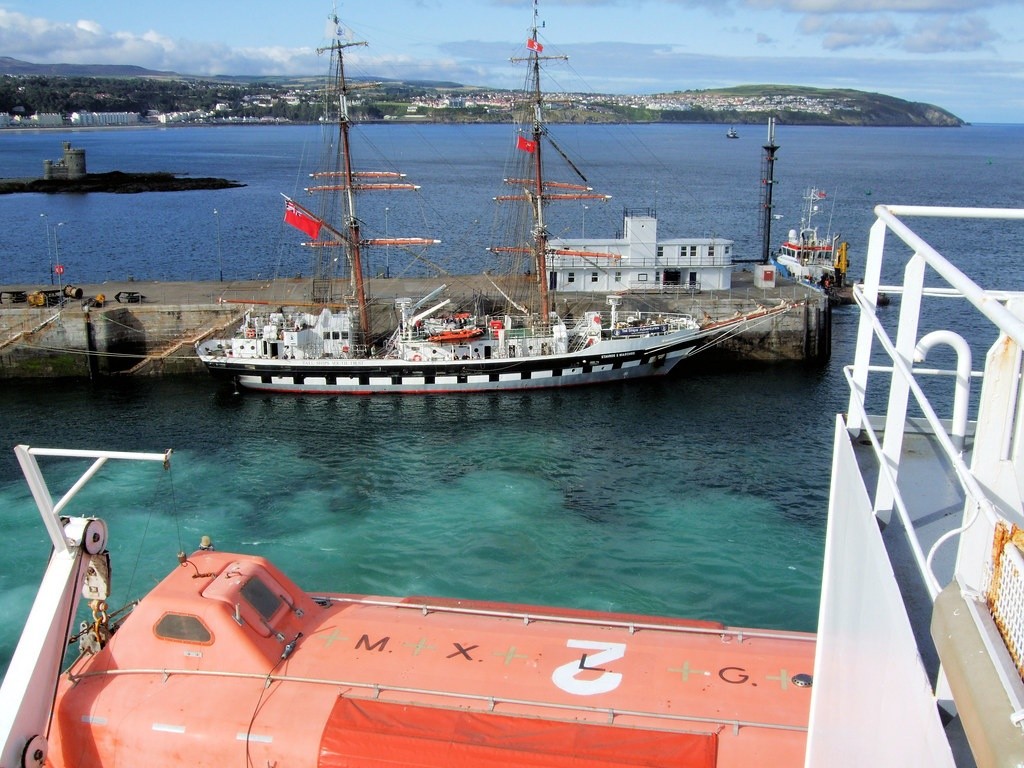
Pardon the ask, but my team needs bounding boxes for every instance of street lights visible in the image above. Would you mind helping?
[385,206,391,278]
[583,204,589,240]
[53,222,67,308]
[213,208,223,283]
[39,213,54,285]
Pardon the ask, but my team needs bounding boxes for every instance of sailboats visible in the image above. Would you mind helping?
[195,1,802,396]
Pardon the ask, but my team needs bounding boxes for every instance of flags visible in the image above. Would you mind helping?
[284,200,323,239]
[516,135,536,152]
[526,37,543,53]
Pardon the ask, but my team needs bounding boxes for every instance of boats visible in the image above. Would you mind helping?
[771,184,892,310]
[726,126,740,140]
